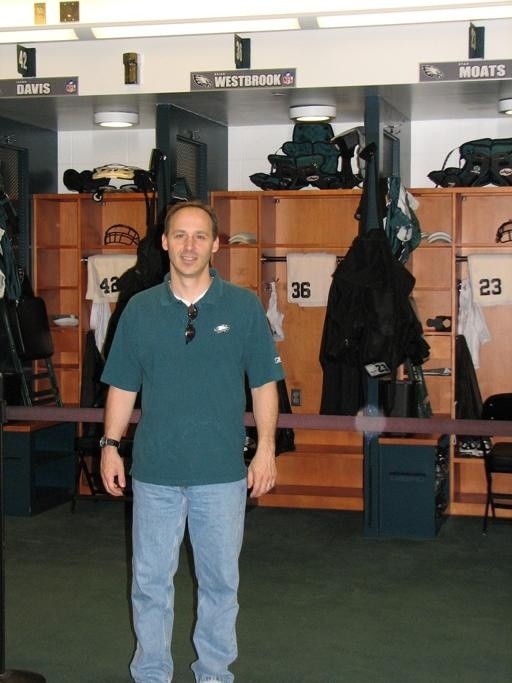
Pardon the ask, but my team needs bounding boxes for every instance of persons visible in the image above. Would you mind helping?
[97,200,287,682]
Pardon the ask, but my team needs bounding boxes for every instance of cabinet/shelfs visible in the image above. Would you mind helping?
[452,188,511,517]
[258,188,452,513]
[33,186,260,410]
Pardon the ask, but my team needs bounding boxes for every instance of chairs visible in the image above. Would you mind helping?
[480,393,511,533]
[1,264,63,408]
[71,397,133,514]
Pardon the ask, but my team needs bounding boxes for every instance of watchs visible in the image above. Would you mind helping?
[99,435,122,450]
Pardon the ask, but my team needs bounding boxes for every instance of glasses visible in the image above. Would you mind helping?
[185,303,198,345]
